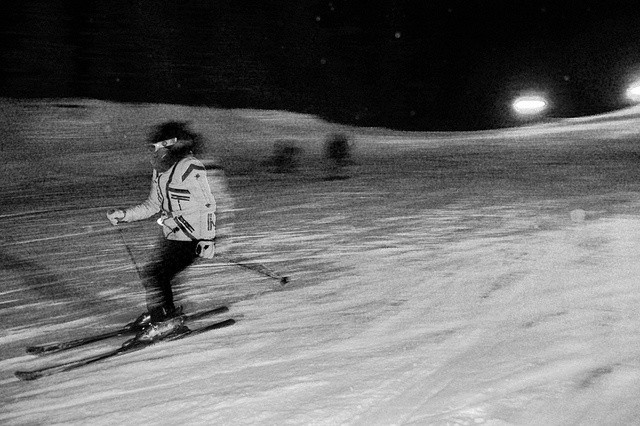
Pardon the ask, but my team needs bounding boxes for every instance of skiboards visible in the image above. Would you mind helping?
[14,305,236,382]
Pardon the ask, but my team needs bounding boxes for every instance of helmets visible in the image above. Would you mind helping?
[145,122,192,172]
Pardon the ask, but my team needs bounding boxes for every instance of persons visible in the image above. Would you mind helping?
[106,121,235,341]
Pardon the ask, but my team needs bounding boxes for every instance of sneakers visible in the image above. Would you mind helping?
[137,316,185,342]
[134,303,177,328]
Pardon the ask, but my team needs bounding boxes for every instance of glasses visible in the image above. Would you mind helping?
[148,137,178,155]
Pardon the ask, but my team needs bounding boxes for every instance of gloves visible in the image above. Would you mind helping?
[107,208,125,226]
[196,239,216,260]
